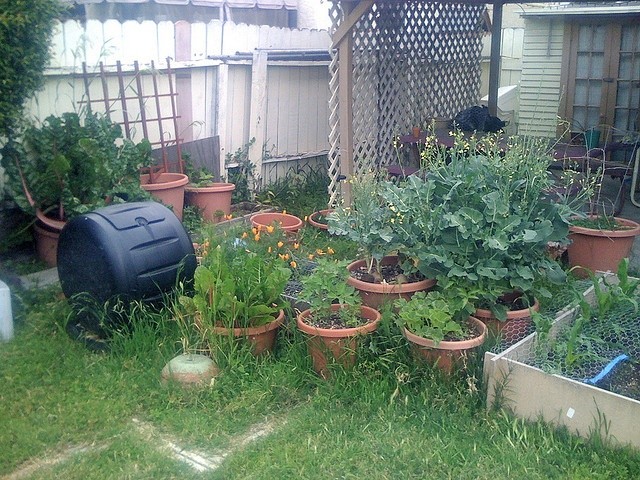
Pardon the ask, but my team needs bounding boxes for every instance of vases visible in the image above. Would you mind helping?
[348,253,436,311]
[464,286,540,343]
[568,212,639,279]
[307,207,346,238]
[250,206,304,240]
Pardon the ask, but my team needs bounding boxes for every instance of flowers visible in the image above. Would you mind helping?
[345,170,437,273]
[393,115,587,304]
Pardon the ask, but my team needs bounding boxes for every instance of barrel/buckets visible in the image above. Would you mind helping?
[57,202,198,335]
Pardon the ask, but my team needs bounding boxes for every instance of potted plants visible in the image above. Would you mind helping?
[180,147,236,227]
[182,243,292,356]
[295,257,381,379]
[6,112,145,269]
[127,136,188,231]
[393,292,485,379]
